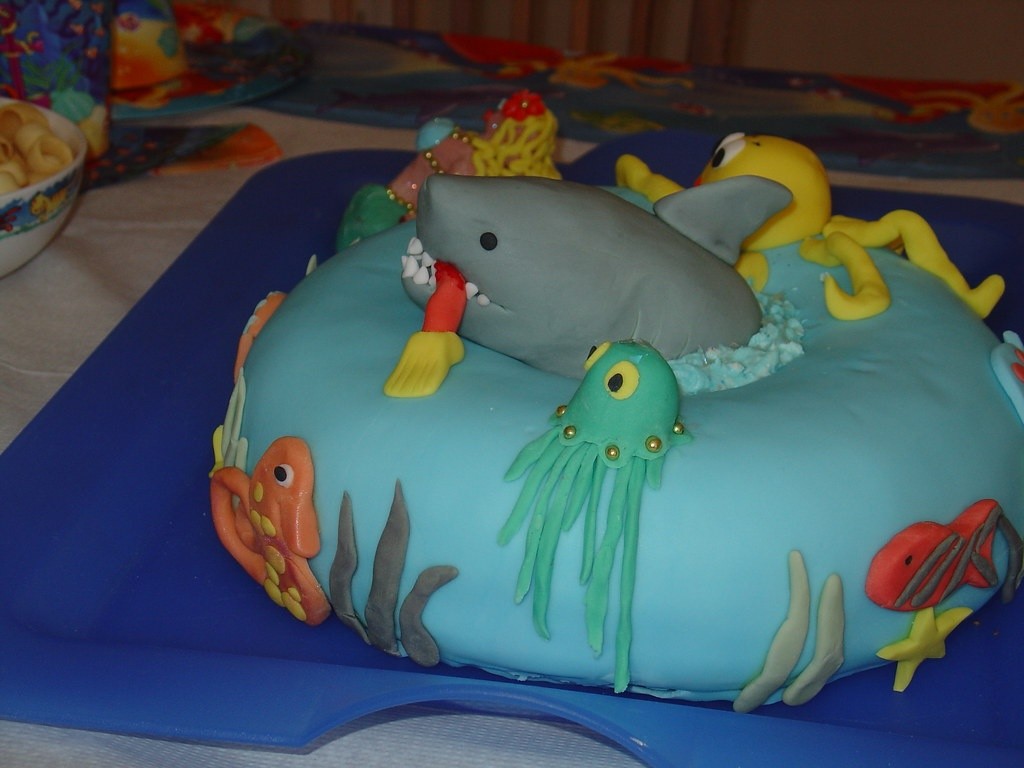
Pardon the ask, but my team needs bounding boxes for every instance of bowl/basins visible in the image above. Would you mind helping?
[0,96,87,279]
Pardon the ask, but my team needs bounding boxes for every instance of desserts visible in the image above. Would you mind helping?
[204,89,1024,711]
